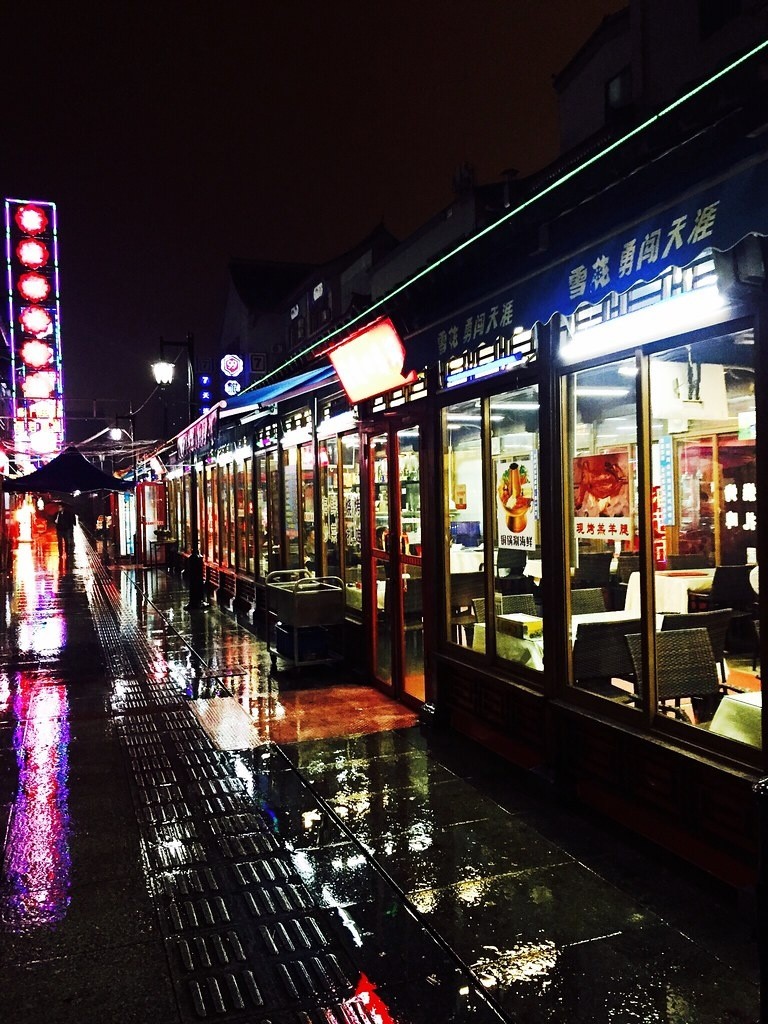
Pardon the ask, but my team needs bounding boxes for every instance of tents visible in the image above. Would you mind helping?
[1,444,143,577]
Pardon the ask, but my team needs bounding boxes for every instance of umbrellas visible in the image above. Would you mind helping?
[46,502,71,516]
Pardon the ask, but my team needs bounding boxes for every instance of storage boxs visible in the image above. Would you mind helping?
[495,613,543,640]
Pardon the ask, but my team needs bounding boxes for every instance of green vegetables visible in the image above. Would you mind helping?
[502,465,528,481]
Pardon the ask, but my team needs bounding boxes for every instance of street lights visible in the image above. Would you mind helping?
[153,333,213,609]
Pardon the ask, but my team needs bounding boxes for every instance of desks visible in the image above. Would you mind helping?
[346,581,386,610]
[709,692,762,749]
[624,568,715,632]
[471,611,664,677]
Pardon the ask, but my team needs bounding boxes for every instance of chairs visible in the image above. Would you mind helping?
[272,538,758,733]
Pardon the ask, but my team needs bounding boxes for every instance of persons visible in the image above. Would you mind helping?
[304,528,362,586]
[375,527,390,566]
[53,503,70,557]
